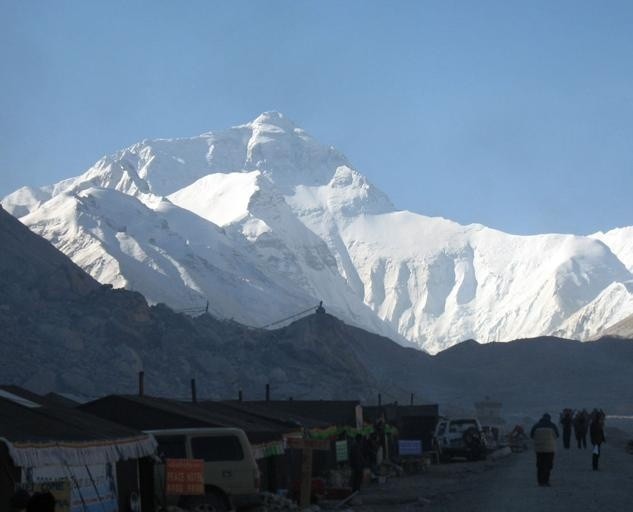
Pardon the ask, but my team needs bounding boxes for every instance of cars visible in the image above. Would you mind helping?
[430,416,489,463]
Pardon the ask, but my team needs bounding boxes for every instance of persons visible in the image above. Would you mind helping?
[530,407,607,487]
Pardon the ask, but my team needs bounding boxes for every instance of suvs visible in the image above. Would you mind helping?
[136,426,262,512]
